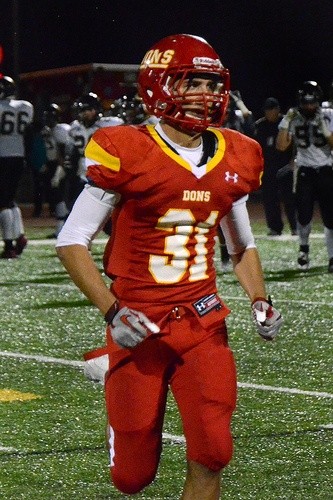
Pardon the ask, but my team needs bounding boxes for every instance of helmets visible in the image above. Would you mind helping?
[0,77,16,99]
[298,81,320,120]
[73,91,100,116]
[136,36,230,132]
[44,103,62,123]
[111,96,136,120]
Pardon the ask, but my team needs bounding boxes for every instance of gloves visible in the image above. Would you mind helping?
[278,107,300,129]
[228,89,249,115]
[314,112,331,137]
[250,299,282,342]
[52,166,65,188]
[104,300,161,348]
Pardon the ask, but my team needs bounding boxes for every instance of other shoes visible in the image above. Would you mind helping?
[267,230,281,235]
[292,226,297,235]
[16,235,27,254]
[48,233,57,238]
[0,248,16,258]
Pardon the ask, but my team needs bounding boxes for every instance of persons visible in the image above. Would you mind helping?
[217,90,258,272]
[37,95,126,239]
[276,81,333,273]
[0,76,34,259]
[52,34,286,500]
[110,81,162,126]
[255,96,299,235]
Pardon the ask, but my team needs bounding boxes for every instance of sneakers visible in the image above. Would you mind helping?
[328,257,333,273]
[298,243,309,264]
[82,348,109,385]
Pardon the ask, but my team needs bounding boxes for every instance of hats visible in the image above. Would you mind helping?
[264,97,279,111]
